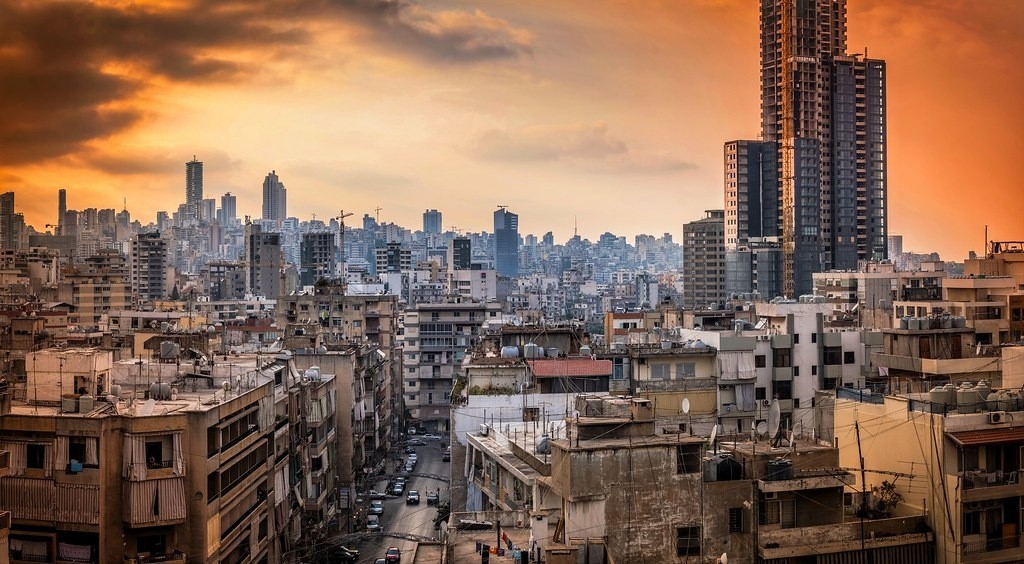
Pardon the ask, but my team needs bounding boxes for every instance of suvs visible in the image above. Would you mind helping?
[370,500,385,516]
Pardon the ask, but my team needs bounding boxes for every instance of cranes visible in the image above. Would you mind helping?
[336,209,355,294]
[375,206,382,227]
[497,204,511,211]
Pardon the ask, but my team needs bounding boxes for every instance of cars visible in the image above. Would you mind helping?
[421,433,442,442]
[330,546,360,558]
[385,547,401,563]
[393,484,404,495]
[406,490,420,504]
[408,426,416,435]
[328,552,356,564]
[396,444,419,489]
[365,514,381,531]
[426,491,439,505]
[442,450,450,462]
[374,558,389,564]
[417,426,426,435]
[407,437,427,446]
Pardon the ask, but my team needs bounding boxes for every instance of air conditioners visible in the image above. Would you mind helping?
[753,335,761,341]
[762,335,772,340]
[990,411,1006,424]
[763,399,771,406]
[480,424,487,435]
